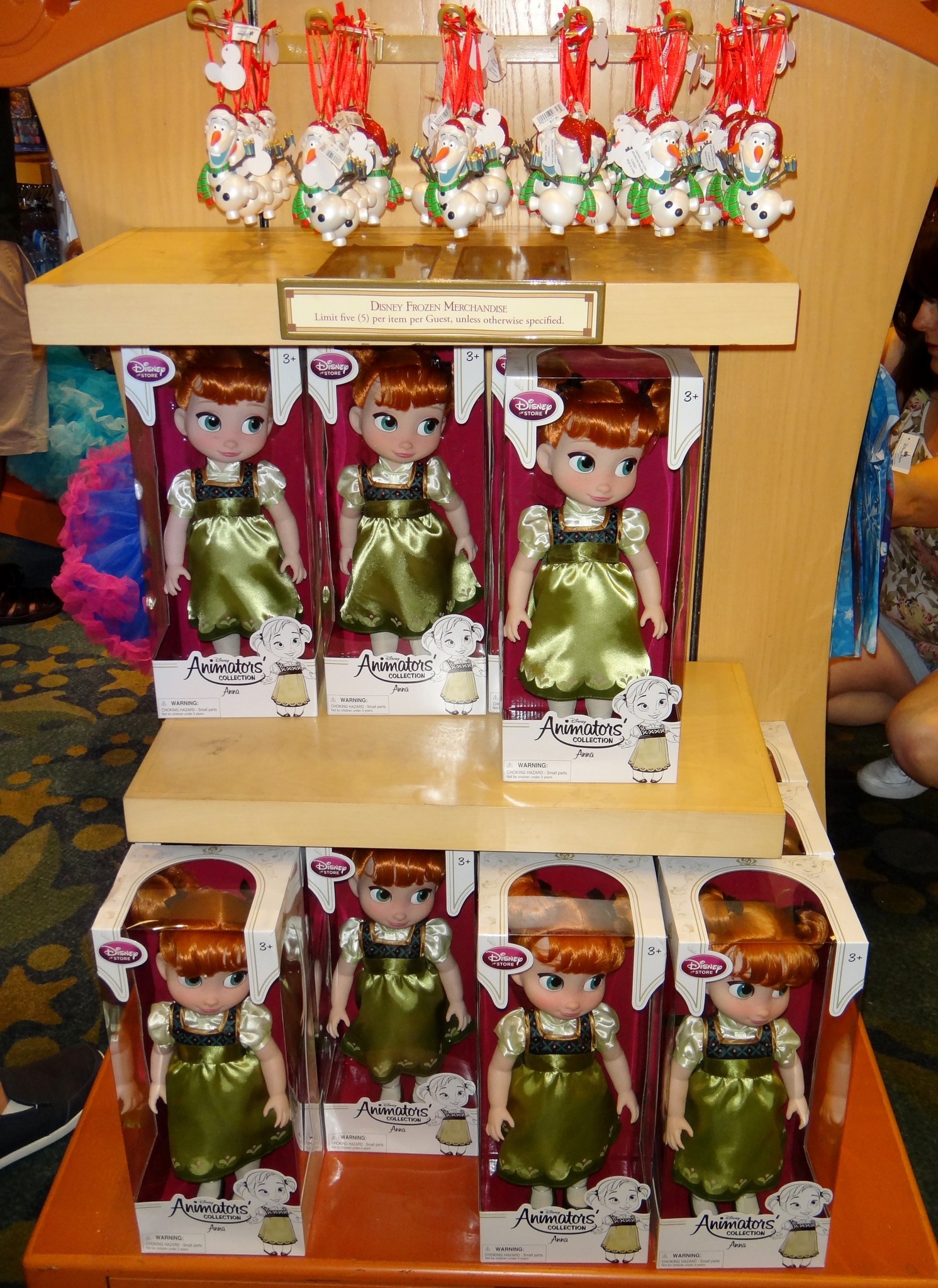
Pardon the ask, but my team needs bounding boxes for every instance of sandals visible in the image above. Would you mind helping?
[0,585,63,626]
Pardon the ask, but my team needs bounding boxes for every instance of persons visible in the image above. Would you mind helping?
[0,1078,83,1171]
[503,376,669,721]
[337,343,481,657]
[164,346,308,661]
[486,875,639,1208]
[664,881,829,1218]
[827,239,938,799]
[0,238,158,663]
[129,868,296,1201]
[326,847,473,1104]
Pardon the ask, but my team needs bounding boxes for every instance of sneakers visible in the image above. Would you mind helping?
[857,755,929,798]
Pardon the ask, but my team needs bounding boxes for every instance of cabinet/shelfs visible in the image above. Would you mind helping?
[0,0,938,1288]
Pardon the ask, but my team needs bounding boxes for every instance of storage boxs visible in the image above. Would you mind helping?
[119,346,324,716]
[303,345,497,719]
[492,349,703,784]
[90,720,868,1268]
